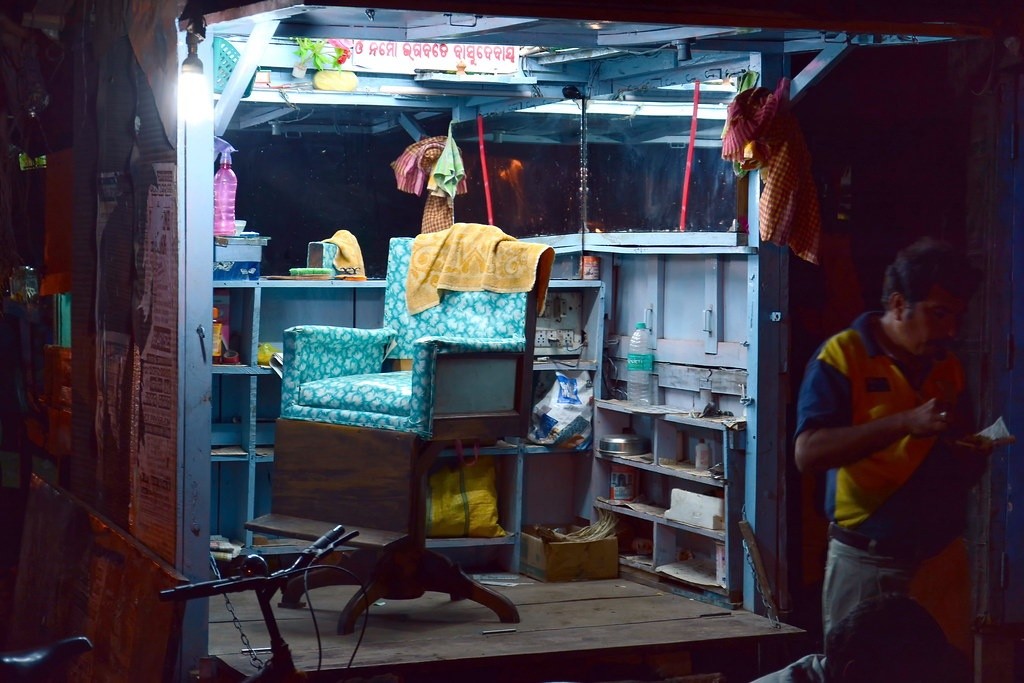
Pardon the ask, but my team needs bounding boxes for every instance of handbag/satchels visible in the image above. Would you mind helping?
[424,439,505,538]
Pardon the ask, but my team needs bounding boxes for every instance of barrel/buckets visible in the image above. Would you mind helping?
[714,539,726,585]
[628,322,652,407]
[580,256,599,280]
[610,463,639,501]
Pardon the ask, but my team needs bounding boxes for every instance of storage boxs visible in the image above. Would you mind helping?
[515,518,621,584]
[664,487,728,531]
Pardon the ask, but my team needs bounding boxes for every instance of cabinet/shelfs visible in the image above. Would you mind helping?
[208,277,608,565]
[585,400,746,597]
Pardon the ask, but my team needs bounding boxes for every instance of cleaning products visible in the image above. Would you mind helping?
[214,136,238,237]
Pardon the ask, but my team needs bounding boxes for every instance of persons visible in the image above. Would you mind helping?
[747,593,947,683]
[792,238,1017,683]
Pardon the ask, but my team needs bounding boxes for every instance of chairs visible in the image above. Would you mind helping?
[234,222,555,631]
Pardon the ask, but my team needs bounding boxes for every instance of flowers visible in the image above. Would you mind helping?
[294,39,352,74]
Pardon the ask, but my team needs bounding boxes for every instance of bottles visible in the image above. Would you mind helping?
[9,266,39,302]
[214,152,237,237]
[213,307,222,365]
[695,439,708,472]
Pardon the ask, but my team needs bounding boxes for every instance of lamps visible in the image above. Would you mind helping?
[181,34,206,73]
[673,39,694,61]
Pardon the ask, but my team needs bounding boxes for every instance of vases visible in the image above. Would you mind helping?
[291,64,306,79]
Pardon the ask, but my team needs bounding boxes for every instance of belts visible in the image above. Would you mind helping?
[829,523,944,553]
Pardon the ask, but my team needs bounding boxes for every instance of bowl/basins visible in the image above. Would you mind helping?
[235,220,247,236]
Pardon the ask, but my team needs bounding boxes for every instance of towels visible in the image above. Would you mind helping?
[320,228,367,282]
[405,222,556,319]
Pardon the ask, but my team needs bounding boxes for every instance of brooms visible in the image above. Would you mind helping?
[528,506,622,543]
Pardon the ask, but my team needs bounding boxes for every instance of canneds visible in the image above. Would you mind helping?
[10,265,38,305]
[579,256,600,280]
[609,462,640,500]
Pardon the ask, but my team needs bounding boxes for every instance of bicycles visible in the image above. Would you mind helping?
[0,525,360,683]
